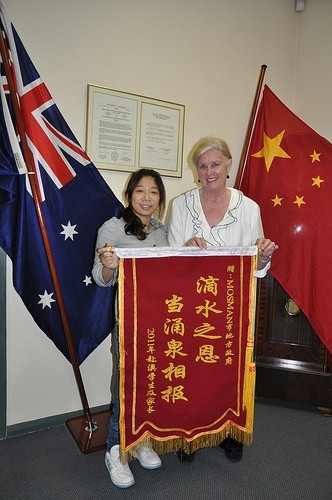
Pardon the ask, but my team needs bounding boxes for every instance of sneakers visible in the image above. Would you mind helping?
[128,442,162,469]
[105,444,135,489]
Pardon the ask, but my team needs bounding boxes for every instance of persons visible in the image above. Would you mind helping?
[92,168,169,488]
[167,137,279,464]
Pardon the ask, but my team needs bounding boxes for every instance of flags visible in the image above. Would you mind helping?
[0,2,126,367]
[239,83,332,354]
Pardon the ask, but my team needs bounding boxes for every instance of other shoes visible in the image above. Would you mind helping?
[177,449,195,463]
[223,436,243,462]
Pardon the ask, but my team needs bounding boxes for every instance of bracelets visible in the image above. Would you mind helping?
[258,251,272,262]
